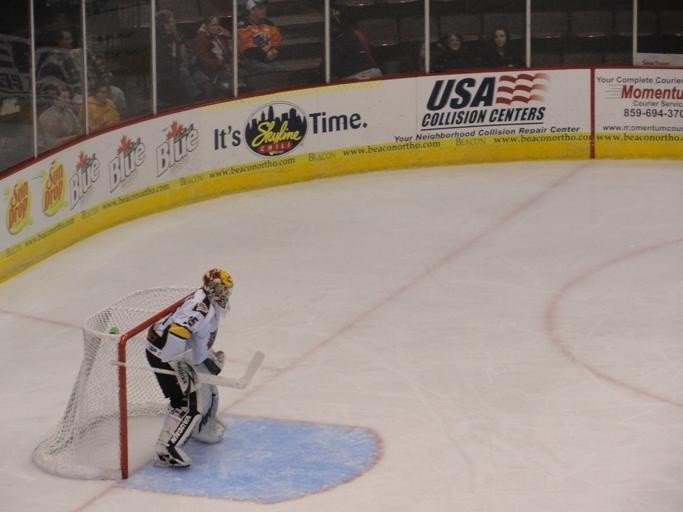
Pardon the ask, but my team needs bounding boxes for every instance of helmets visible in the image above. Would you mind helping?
[201,267,234,301]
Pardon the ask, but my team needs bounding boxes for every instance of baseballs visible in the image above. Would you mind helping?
[110,327,119,334]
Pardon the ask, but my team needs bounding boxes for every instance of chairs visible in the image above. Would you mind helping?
[356,10,683,56]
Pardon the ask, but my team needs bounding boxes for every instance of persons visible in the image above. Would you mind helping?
[142,266,236,469]
[475,26,514,67]
[429,30,481,71]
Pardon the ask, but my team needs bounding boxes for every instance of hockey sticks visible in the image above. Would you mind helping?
[110,351,264,389]
